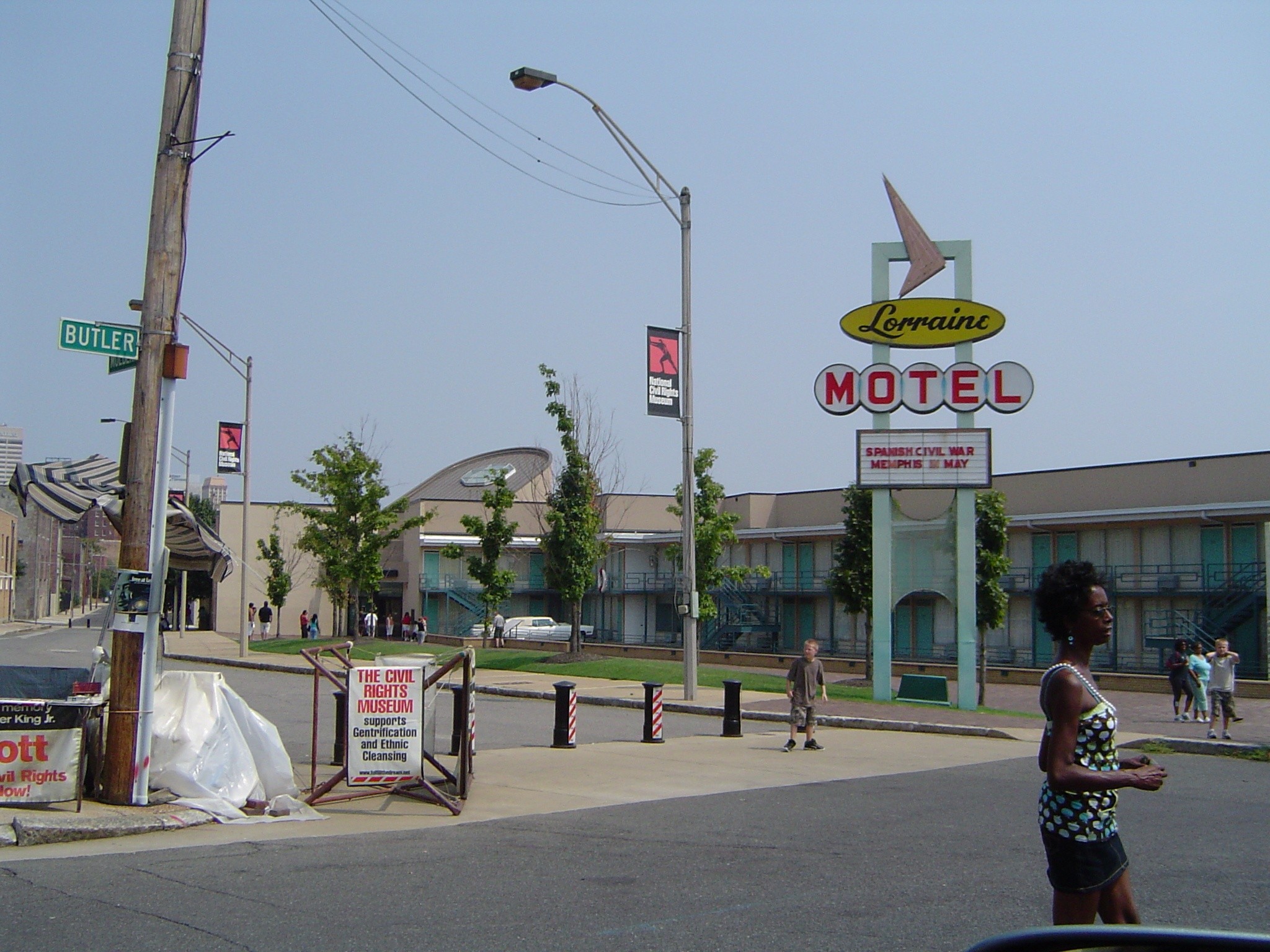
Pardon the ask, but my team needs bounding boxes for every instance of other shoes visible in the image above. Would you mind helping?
[1193,717,1204,723]
[1205,717,1211,723]
[1182,712,1192,722]
[1173,715,1182,722]
[1222,730,1232,740]
[1207,729,1217,739]
[1232,717,1243,722]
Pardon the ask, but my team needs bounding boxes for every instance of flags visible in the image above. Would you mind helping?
[217,421,243,473]
[168,490,183,502]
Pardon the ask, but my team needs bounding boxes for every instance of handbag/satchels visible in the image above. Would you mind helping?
[1166,651,1180,670]
[307,625,311,631]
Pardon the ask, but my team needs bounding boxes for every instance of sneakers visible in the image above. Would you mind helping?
[783,739,796,753]
[804,738,826,751]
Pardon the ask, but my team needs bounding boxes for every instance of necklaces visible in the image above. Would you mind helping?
[1041,663,1116,711]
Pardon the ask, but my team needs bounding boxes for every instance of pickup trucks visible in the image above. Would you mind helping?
[489,616,594,643]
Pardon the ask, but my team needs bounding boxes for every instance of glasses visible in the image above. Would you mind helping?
[1197,647,1203,649]
[1082,605,1116,621]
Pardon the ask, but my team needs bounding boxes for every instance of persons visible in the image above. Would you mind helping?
[386,614,394,641]
[1167,639,1194,722]
[1206,639,1244,740]
[783,639,828,752]
[364,610,378,637]
[1188,642,1211,722]
[259,601,273,639]
[300,610,309,638]
[1037,561,1167,926]
[248,603,257,640]
[159,605,209,635]
[401,612,427,643]
[309,614,321,639]
[492,612,505,648]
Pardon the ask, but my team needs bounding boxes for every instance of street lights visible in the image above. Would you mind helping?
[100,418,192,639]
[511,68,700,700]
[128,297,250,660]
